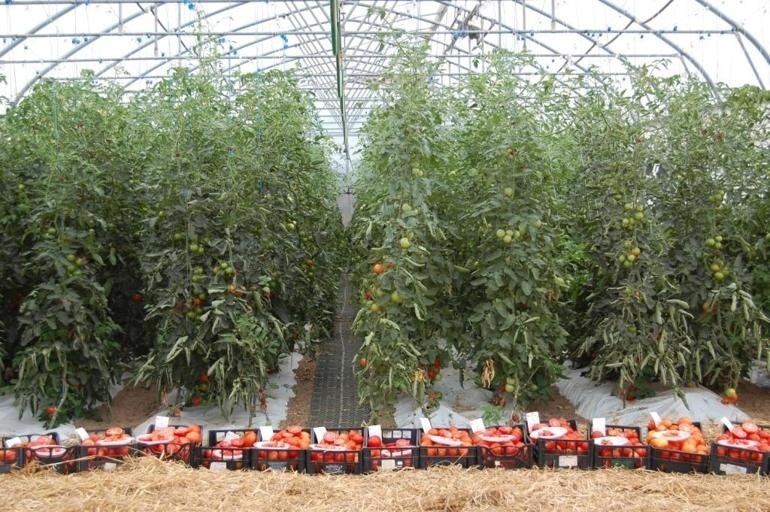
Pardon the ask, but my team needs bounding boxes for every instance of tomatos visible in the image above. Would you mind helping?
[0,167,770,468]
